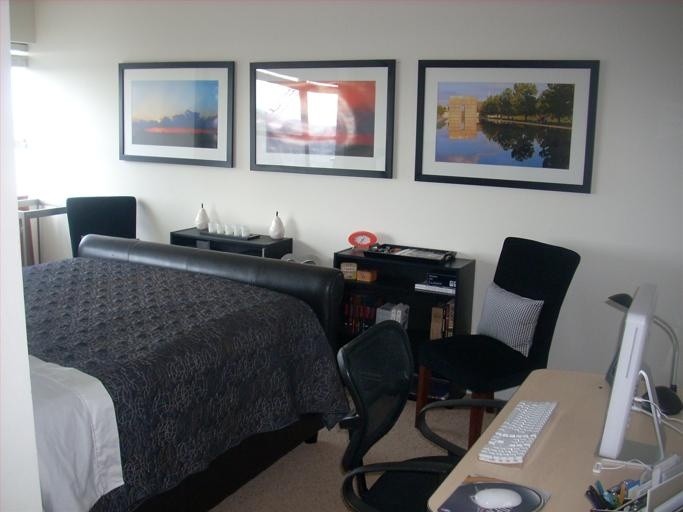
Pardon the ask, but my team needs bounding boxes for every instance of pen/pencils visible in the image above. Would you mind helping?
[585,480,625,510]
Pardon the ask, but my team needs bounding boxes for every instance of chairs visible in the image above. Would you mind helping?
[418,236,579,450]
[336,319,506,512]
[68,197,139,257]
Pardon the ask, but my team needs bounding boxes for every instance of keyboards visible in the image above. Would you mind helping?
[478,400,559,469]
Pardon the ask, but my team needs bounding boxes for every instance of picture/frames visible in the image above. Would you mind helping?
[117,60,236,168]
[414,59,602,194]
[249,60,395,179]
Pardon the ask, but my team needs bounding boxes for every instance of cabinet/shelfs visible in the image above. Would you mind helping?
[168,226,293,259]
[332,243,476,409]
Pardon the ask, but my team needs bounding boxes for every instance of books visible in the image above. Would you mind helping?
[343,292,410,338]
[414,271,457,294]
[429,299,455,341]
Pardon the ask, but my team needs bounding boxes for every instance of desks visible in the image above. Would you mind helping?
[428,369,682,512]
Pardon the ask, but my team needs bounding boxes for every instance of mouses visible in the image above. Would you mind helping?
[475,488,522,509]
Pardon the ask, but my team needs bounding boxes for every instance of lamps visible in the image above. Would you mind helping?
[609,294,683,416]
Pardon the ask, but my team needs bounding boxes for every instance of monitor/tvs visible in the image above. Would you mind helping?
[593,283,666,467]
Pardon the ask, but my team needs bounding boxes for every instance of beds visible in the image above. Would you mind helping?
[22,257,355,511]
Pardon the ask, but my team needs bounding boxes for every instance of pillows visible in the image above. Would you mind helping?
[477,281,543,357]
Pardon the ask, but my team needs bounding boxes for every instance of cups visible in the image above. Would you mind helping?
[208,219,249,236]
